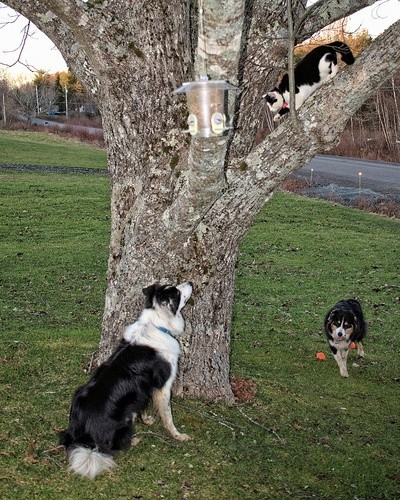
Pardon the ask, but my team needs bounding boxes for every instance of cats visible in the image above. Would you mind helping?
[262,40,356,121]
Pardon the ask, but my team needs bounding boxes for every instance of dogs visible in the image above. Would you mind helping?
[59,280,196,479]
[323,299,367,378]
